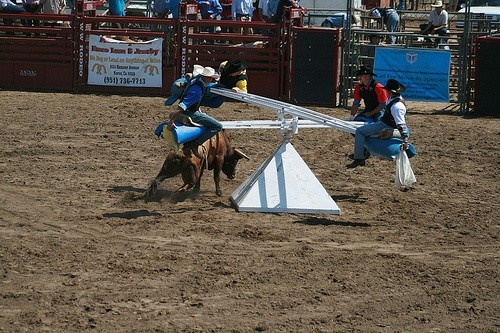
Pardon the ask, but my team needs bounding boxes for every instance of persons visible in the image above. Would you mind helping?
[168,65,223,160]
[369,7,400,44]
[347,70,389,163]
[320,10,360,31]
[0,0,126,38]
[194,0,223,40]
[187,57,248,107]
[420,0,449,48]
[231,0,256,44]
[257,0,293,39]
[369,34,381,45]
[344,78,415,168]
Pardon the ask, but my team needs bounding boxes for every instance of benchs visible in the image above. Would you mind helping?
[404,27,450,37]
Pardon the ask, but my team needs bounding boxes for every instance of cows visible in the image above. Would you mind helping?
[142,127,251,197]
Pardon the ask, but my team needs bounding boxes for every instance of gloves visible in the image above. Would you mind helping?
[344,115,354,121]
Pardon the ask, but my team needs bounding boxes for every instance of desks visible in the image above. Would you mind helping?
[360,16,382,29]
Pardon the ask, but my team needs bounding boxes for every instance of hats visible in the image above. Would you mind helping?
[368,34,384,39]
[193,65,220,79]
[224,58,247,73]
[369,8,375,14]
[381,79,406,93]
[352,14,361,24]
[431,1,443,7]
[355,67,377,77]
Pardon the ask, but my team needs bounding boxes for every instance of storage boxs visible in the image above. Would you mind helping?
[361,0,391,10]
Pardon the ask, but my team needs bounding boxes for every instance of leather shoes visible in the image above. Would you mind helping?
[346,160,365,167]
[184,141,201,159]
[348,152,370,160]
